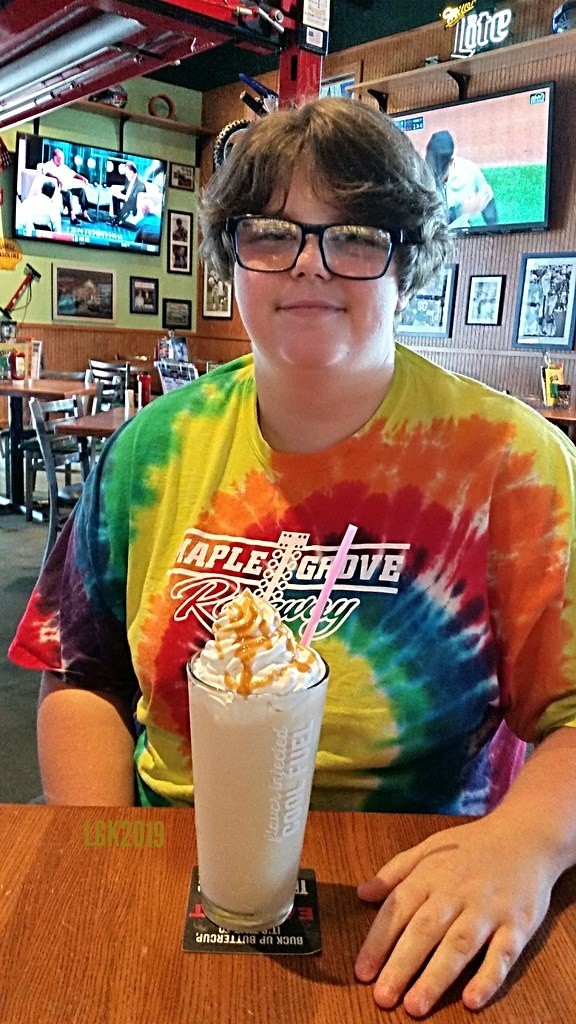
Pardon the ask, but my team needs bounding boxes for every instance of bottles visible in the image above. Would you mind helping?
[0,350,9,379]
[124,386,135,421]
[9,349,25,380]
[137,371,152,411]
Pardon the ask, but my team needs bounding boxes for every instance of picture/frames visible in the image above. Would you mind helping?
[320,61,362,102]
[129,276,160,315]
[465,274,507,326]
[170,161,195,192]
[51,263,117,323]
[162,298,192,329]
[396,263,459,338]
[203,260,233,320]
[511,251,576,351]
[168,209,194,275]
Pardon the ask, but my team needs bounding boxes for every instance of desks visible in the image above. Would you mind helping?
[521,399,576,447]
[0,803,576,1024]
[0,378,97,525]
[55,407,149,526]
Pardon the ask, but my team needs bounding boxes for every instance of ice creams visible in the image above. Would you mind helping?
[190,588,326,722]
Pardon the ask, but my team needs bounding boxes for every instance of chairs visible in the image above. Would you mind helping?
[0,354,132,569]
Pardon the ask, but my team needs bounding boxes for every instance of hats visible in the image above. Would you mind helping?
[426,130,454,182]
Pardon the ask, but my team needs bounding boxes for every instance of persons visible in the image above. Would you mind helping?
[173,218,188,241]
[133,290,154,312]
[208,271,228,310]
[132,205,162,245]
[16,181,62,232]
[106,161,146,226]
[172,246,187,269]
[7,96,576,1021]
[42,149,95,225]
[426,130,498,228]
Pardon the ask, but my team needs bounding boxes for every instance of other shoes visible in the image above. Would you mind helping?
[84,215,94,222]
[70,218,80,225]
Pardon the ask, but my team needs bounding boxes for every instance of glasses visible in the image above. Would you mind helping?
[223,213,407,280]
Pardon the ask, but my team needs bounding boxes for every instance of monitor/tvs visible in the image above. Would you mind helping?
[379,80,554,241]
[12,132,167,255]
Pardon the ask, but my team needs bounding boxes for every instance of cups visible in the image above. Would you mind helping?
[185,643,331,937]
[557,384,570,409]
